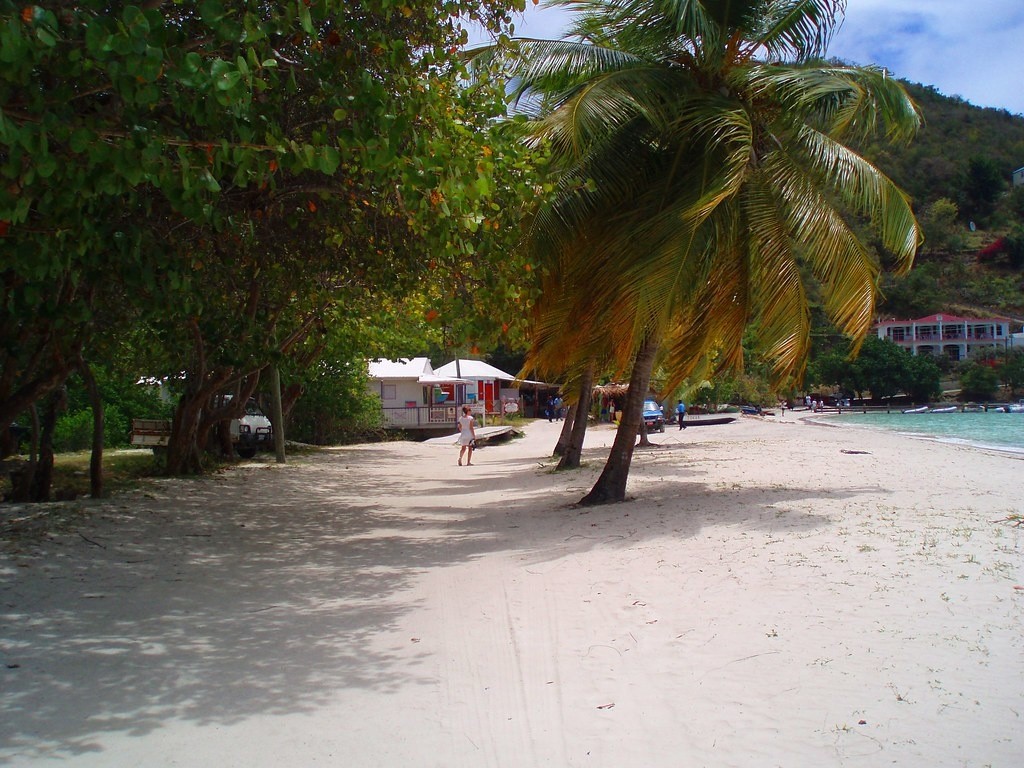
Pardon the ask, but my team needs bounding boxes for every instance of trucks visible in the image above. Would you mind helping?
[129,394,274,461]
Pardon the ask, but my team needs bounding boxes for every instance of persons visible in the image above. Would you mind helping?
[544,391,565,423]
[678,400,686,430]
[599,395,623,423]
[688,402,709,415]
[782,401,785,416]
[457,405,476,466]
[806,393,824,413]
[519,392,535,418]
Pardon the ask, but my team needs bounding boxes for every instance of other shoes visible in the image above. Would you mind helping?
[459,458,462,466]
[467,462,474,466]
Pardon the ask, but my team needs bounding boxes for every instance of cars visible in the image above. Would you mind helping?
[638,400,666,434]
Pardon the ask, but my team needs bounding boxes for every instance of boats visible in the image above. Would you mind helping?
[930,406,958,413]
[904,406,928,414]
